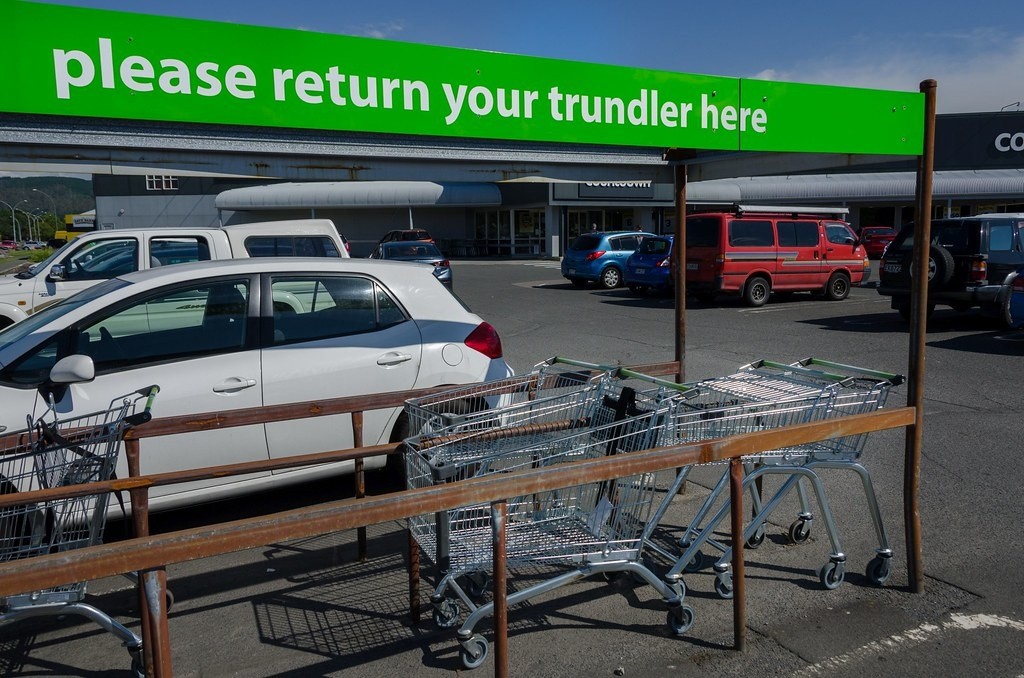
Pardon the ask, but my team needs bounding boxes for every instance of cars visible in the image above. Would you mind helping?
[561,232,674,295]
[0,257,516,551]
[368,230,452,294]
[861,227,898,259]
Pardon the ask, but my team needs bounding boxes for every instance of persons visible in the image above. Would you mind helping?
[590,223,598,233]
[637,224,644,245]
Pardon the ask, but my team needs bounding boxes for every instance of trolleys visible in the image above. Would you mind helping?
[403,357,906,668]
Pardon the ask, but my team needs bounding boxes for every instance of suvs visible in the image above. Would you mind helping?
[876,218,1024,321]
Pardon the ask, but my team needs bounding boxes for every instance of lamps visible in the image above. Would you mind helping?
[120,208,124,213]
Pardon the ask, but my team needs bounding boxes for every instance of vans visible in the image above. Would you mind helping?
[0,384,161,678]
[0,219,351,333]
[670,213,871,307]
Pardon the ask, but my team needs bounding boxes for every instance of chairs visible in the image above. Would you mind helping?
[417,247,426,255]
[203,287,245,350]
